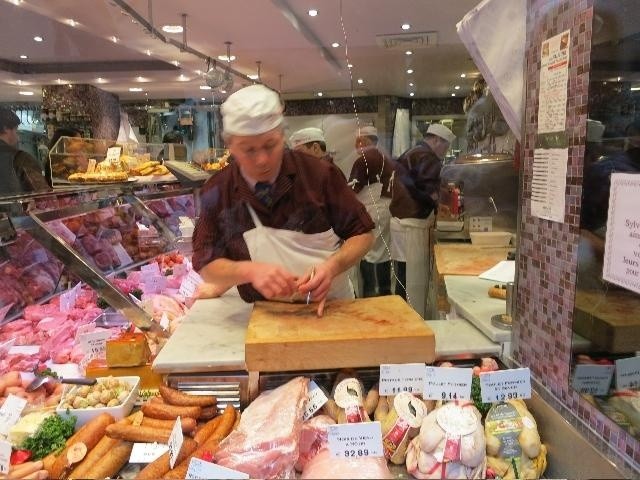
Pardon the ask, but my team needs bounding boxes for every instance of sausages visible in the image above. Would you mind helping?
[43,386,241,480]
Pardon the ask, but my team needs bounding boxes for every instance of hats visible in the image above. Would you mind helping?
[426,122,457,144]
[219,83,285,138]
[288,127,325,149]
[355,126,378,137]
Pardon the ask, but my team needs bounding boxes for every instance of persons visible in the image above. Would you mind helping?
[1,106,186,262]
[190,83,375,316]
[187,281,232,308]
[288,126,366,299]
[388,124,457,318]
[345,124,392,298]
[574,118,640,291]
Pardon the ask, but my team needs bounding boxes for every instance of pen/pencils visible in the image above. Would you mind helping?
[307,264,314,304]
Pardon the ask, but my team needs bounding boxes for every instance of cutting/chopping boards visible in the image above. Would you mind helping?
[572,287,639,357]
[243,294,436,373]
[435,241,520,276]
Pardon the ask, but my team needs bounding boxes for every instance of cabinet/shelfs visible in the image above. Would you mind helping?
[0,183,202,390]
[49,136,187,187]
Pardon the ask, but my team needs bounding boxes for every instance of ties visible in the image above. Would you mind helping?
[255,181,271,199]
